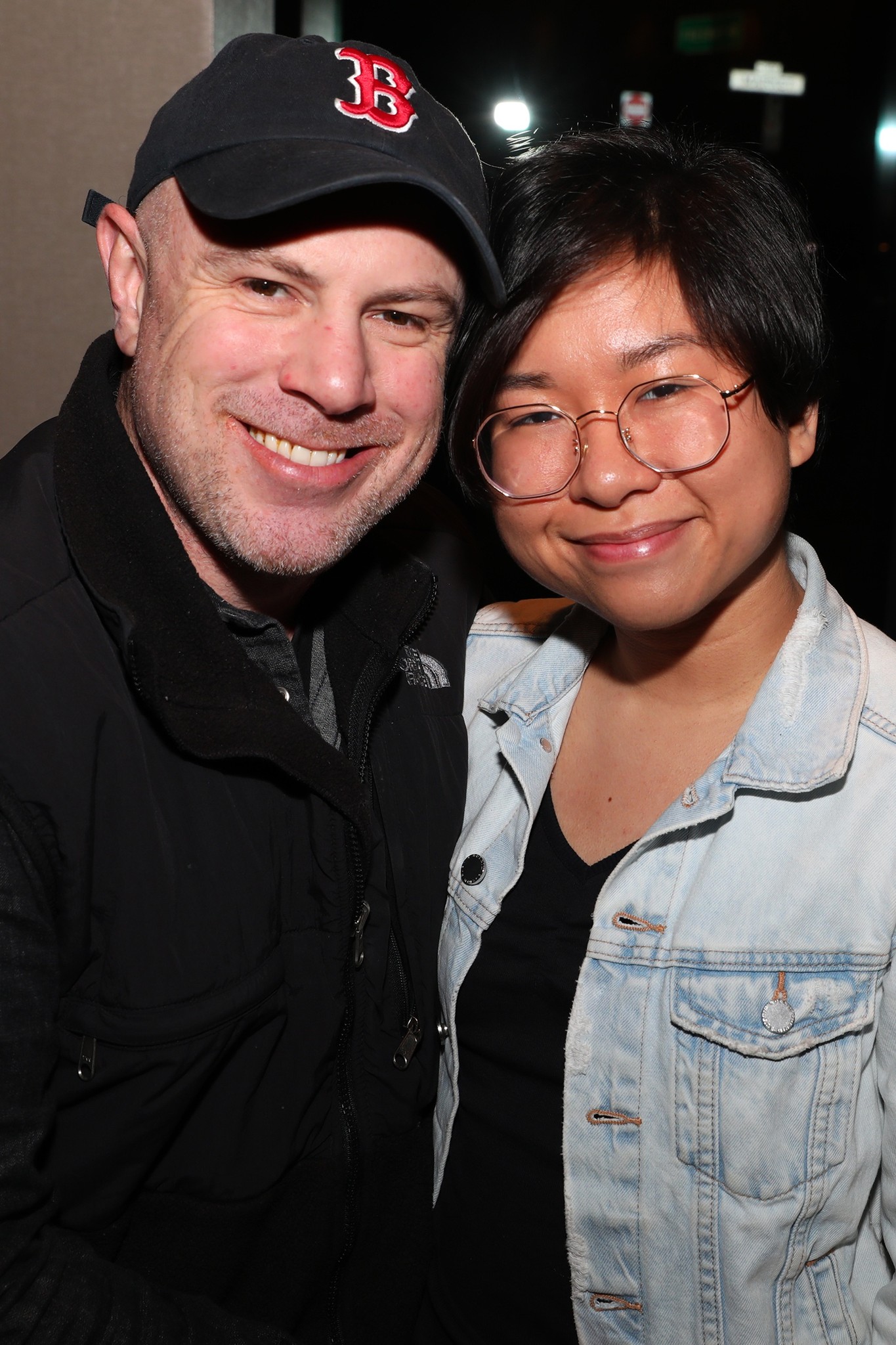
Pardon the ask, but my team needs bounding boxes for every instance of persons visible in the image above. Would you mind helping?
[0,30,506,1345]
[421,134,896,1345]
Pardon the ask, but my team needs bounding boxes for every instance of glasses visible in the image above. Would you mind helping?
[468,375,757,499]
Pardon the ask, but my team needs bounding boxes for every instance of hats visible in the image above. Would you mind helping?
[82,32,506,308]
